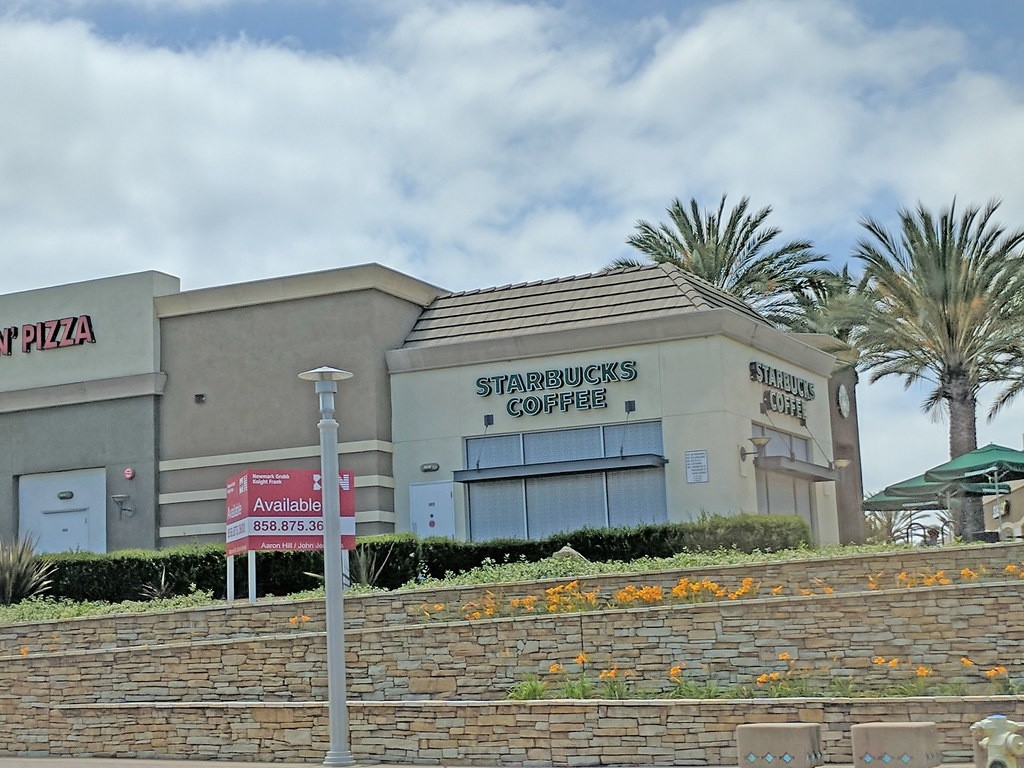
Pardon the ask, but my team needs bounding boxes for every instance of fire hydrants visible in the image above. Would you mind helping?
[969,713,1024,768]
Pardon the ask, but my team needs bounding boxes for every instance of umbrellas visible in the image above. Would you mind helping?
[885,472,1011,542]
[924,439,1023,544]
[861,490,948,546]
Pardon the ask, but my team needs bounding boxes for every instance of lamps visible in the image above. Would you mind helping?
[111,494,136,522]
[738,436,771,478]
[823,458,853,496]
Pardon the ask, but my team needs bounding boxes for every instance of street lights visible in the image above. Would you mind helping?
[297,364,355,768]
